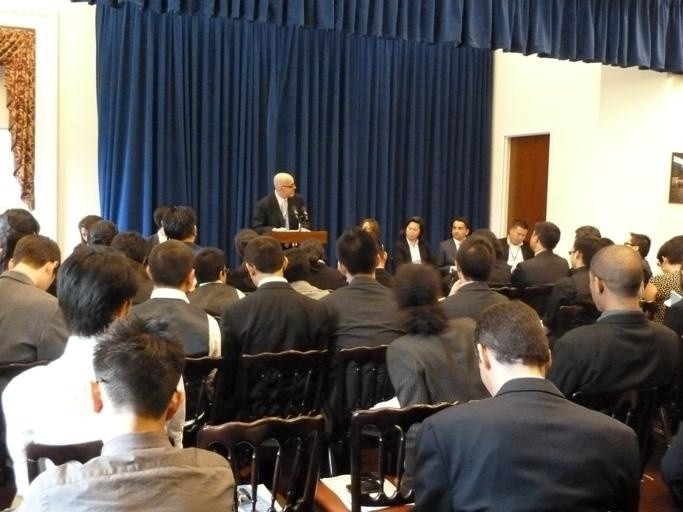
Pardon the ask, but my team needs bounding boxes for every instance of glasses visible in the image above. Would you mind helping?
[624,242,632,247]
[283,185,294,189]
[569,250,579,254]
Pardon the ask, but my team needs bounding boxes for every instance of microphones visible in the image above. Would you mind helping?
[291,206,299,220]
[301,206,309,221]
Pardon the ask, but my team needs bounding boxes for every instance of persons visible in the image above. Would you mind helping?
[386,263,488,409]
[220,238,328,409]
[252,172,309,233]
[0,207,682,512]
